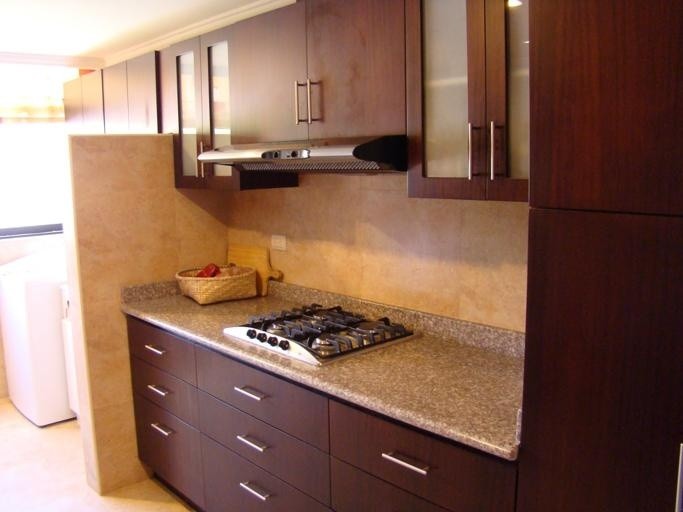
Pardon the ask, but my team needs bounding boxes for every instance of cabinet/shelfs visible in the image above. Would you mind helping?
[516,0,683,511]
[127,317,517,512]
[64,0,529,202]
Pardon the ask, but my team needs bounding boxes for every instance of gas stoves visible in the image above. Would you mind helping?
[223,301,422,366]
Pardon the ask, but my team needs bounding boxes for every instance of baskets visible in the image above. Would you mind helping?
[175,264,256,305]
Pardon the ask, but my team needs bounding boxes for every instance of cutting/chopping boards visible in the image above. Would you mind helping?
[227,244,282,297]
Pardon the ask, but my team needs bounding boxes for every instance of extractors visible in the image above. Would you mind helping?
[197,135,409,175]
